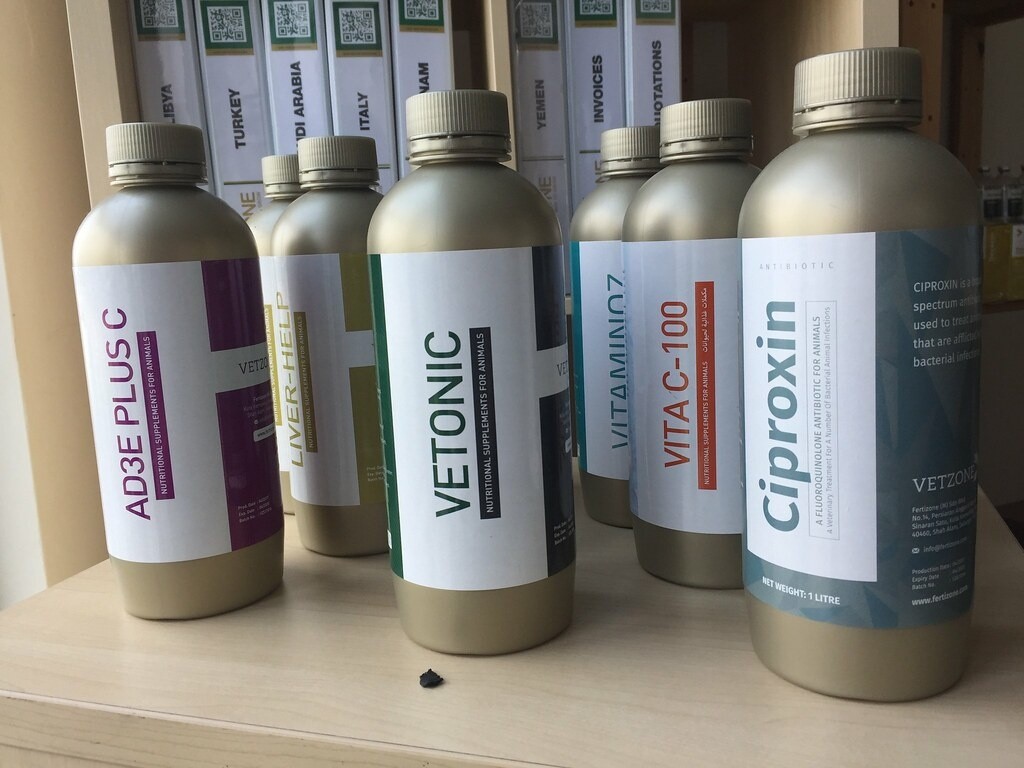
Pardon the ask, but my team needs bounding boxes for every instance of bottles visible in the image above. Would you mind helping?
[739,48,982,703]
[270,136,427,558]
[365,89,576,656]
[244,153,309,517]
[568,127,664,529]
[70,121,285,621]
[621,99,766,593]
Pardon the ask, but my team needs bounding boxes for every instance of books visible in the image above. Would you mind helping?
[125,0,684,234]
